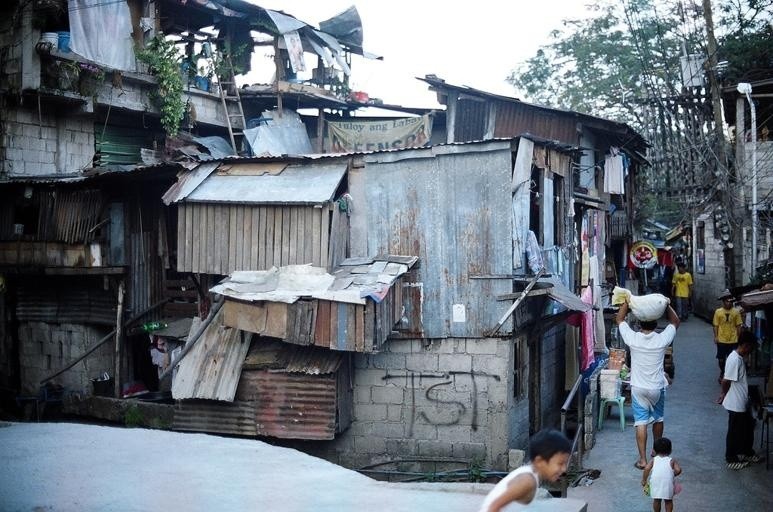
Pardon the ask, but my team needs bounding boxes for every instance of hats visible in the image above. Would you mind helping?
[717,289,732,300]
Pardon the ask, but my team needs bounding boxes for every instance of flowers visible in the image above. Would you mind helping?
[79,62,106,81]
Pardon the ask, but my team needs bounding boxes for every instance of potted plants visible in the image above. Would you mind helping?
[134,32,248,137]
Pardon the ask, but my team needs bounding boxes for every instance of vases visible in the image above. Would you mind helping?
[80,77,98,97]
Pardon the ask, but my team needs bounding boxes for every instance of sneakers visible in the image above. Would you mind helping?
[741,455,764,464]
[725,461,749,470]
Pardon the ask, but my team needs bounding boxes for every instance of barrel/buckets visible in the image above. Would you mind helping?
[601,369,623,399]
[608,349,626,369]
[91,375,113,398]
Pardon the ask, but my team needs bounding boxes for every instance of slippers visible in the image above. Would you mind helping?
[635,462,644,470]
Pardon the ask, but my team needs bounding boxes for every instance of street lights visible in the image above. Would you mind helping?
[735,80,760,371]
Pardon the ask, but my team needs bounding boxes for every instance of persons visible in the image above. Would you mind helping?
[711,295,743,384]
[614,296,681,471]
[637,436,682,512]
[478,428,573,512]
[711,330,765,472]
[670,262,694,321]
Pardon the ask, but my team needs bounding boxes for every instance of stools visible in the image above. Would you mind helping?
[597,396,626,432]
